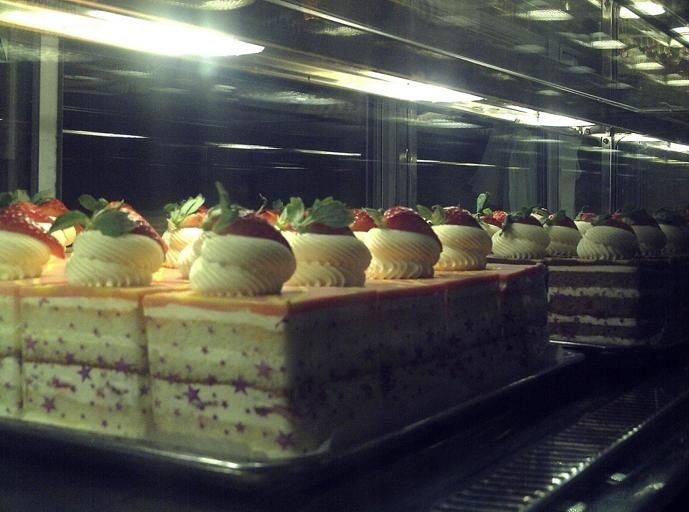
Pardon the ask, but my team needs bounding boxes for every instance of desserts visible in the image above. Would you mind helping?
[1,191,689,464]
